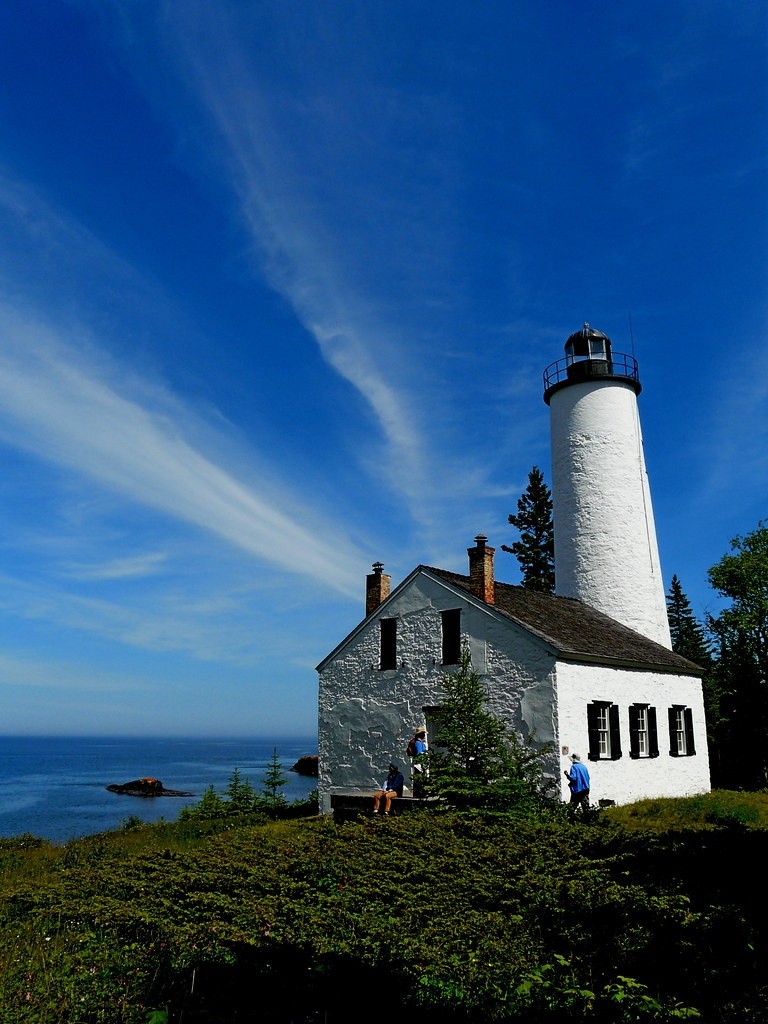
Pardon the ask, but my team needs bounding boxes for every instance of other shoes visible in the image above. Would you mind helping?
[373,809,379,814]
[385,811,389,816]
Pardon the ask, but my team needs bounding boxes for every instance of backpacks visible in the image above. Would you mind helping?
[407,738,418,757]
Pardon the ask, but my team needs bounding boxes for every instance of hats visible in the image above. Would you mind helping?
[567,752,583,764]
[388,760,399,771]
[413,728,426,736]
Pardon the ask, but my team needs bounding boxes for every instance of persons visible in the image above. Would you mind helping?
[564,753,590,812]
[373,764,404,815]
[406,727,429,798]
